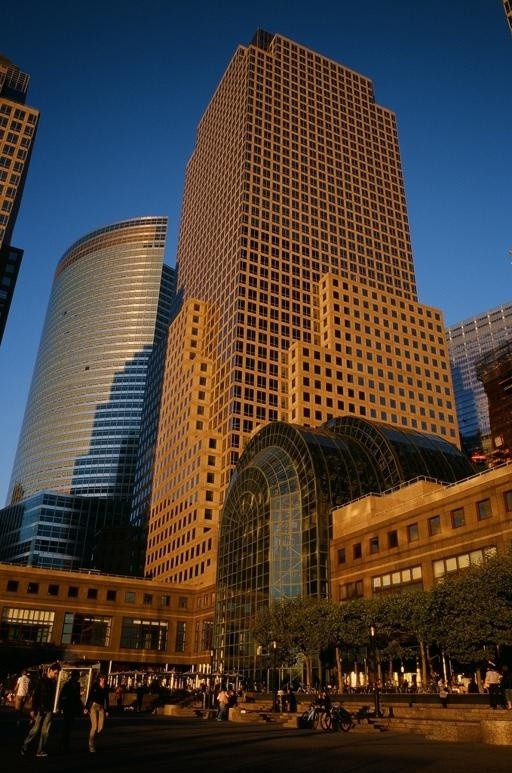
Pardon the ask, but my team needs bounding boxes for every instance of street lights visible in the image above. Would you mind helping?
[369,620,383,718]
[207,647,214,708]
[271,637,278,711]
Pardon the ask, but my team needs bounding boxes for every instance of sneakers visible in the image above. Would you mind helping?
[35,752,47,758]
[20,746,27,756]
[89,745,96,753]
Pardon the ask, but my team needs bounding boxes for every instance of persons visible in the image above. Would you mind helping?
[13,668,32,726]
[116,668,508,723]
[84,671,110,755]
[15,663,63,762]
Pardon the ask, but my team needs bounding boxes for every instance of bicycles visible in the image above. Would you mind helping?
[298,699,351,731]
